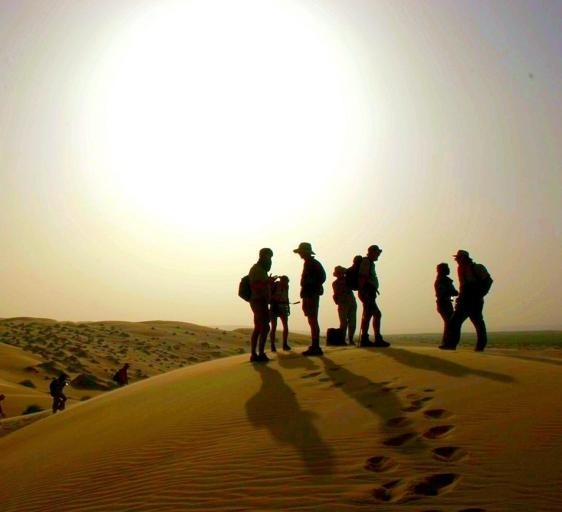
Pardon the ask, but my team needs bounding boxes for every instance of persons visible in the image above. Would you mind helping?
[49,372,72,413]
[117,362,131,386]
[293,242,326,357]
[434,262,459,349]
[0,393,7,419]
[332,266,357,345]
[355,245,392,348]
[247,247,277,362]
[270,275,291,352]
[437,249,494,352]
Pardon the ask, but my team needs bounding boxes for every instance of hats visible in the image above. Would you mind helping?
[293,243,315,255]
[333,265,347,276]
[452,250,472,260]
[366,245,382,256]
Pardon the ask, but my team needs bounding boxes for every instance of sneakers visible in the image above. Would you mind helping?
[250,334,485,361]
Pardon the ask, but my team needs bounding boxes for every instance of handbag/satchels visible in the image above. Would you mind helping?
[238,275,251,301]
